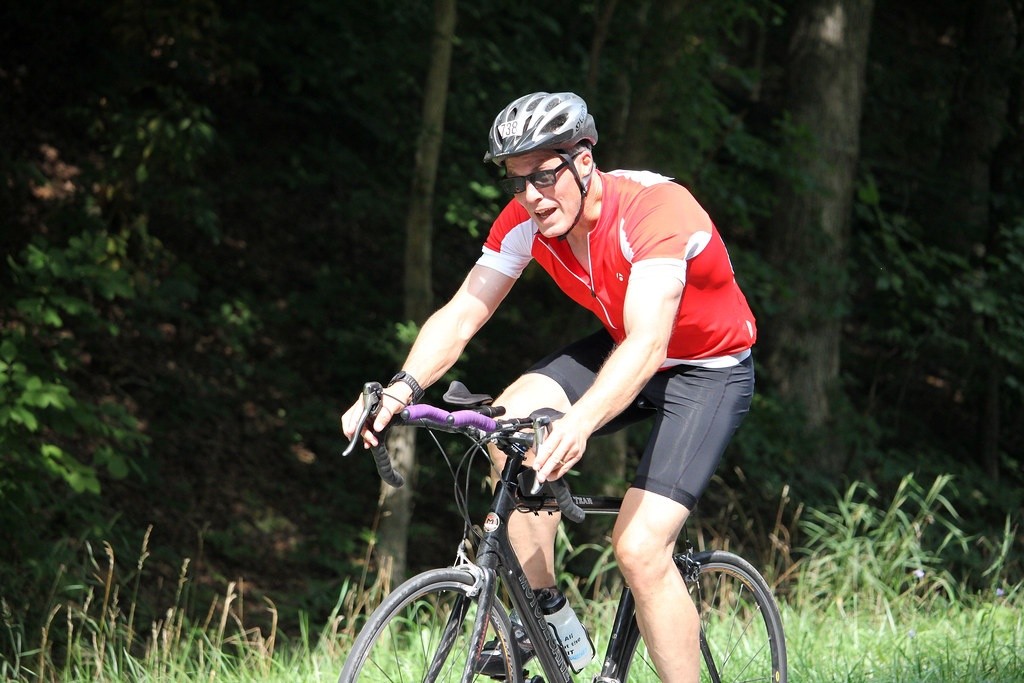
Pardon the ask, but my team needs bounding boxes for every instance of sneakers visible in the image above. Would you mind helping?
[473,603,536,675]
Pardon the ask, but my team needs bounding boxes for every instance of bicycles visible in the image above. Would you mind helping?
[337,381,787,683]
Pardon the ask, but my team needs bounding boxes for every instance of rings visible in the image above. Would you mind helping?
[559,460,565,465]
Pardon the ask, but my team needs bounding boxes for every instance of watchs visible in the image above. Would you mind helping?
[387,371,425,404]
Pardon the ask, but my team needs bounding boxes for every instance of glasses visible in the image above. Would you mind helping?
[498,147,588,195]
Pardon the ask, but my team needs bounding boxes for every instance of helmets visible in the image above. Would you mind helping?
[483,93,598,166]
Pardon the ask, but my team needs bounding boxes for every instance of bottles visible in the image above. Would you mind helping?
[537,587,594,670]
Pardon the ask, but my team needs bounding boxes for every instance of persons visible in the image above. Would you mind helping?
[341,91,757,683]
